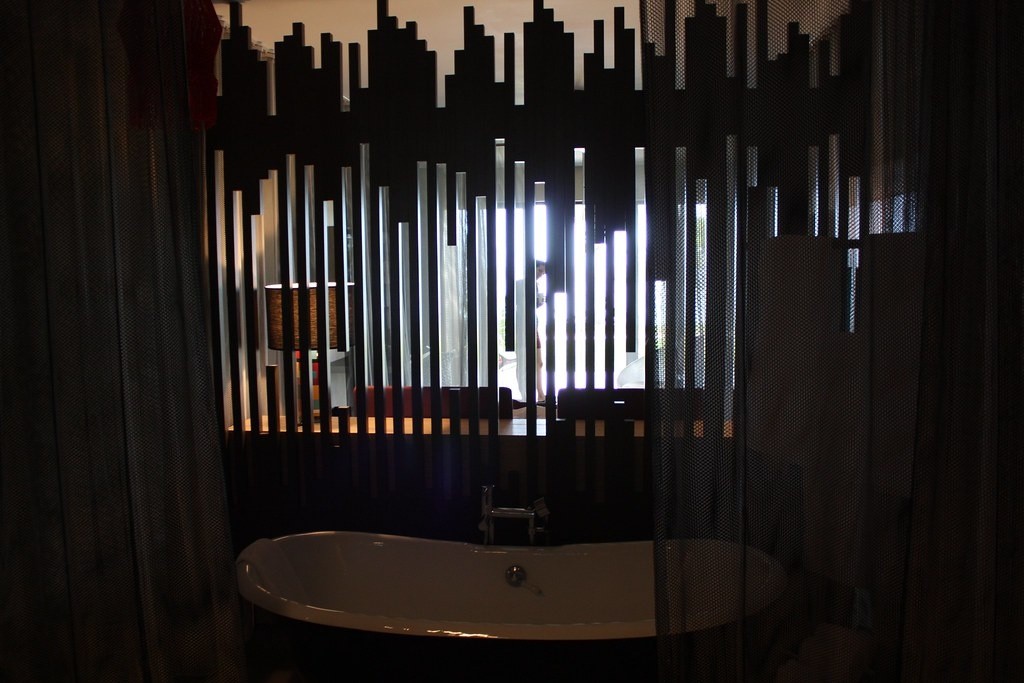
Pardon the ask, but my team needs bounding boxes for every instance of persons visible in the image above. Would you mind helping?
[502,257,559,405]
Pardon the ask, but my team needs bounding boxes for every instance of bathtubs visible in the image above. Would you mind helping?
[233,528,790,683]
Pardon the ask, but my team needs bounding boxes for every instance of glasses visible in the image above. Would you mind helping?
[537,268,545,275]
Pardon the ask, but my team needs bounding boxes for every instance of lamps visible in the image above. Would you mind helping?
[252,281,356,434]
